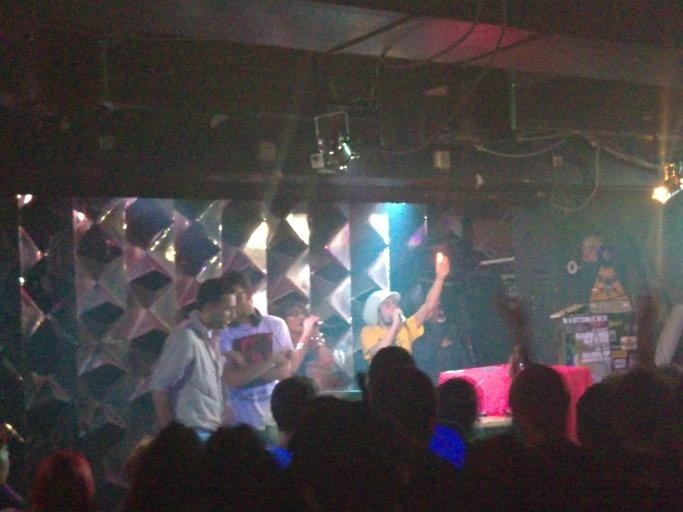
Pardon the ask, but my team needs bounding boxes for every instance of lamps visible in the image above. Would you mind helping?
[650,159,683,206]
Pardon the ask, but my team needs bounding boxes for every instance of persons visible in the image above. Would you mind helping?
[146,279,234,438]
[361,253,452,365]
[28,448,95,511]
[0,422,28,512]
[128,285,683,512]
[212,267,293,440]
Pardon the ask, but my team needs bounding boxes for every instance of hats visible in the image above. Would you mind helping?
[363,290,401,326]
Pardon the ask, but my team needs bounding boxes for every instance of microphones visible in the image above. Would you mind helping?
[400,314,406,323]
[301,320,324,325]
